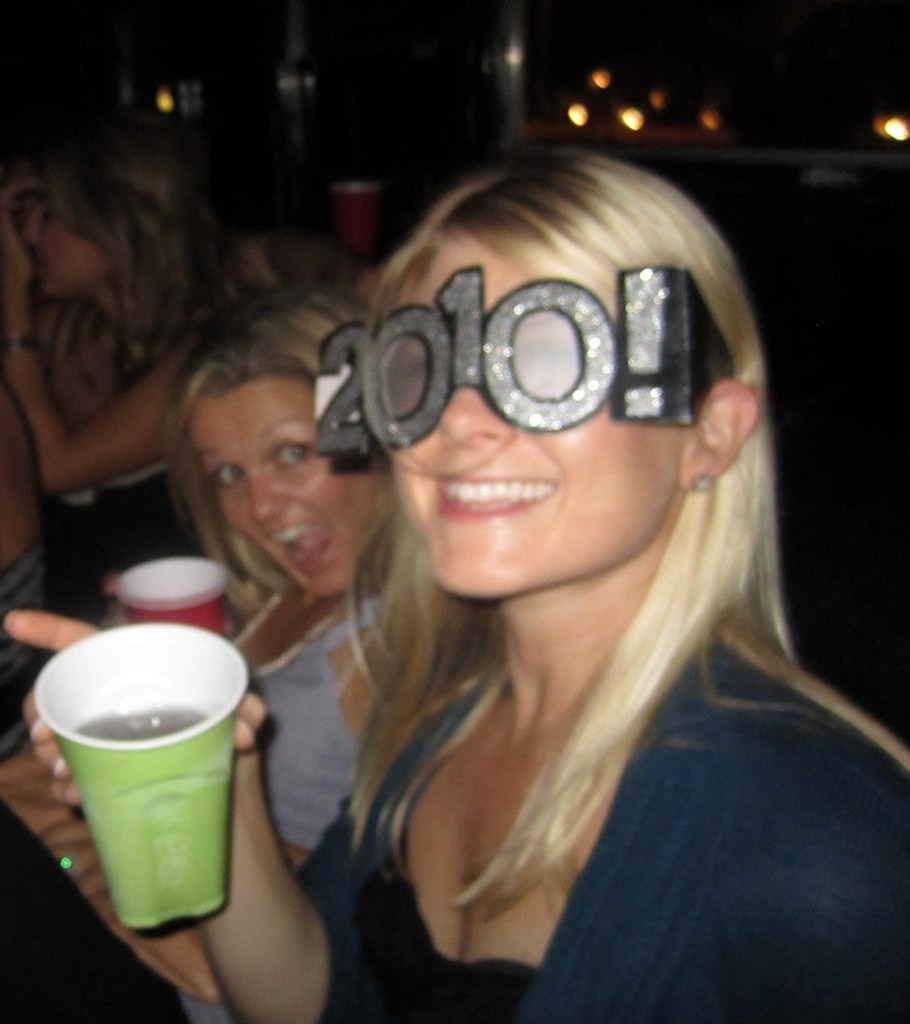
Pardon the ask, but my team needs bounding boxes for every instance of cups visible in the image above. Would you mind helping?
[33,622,249,928]
[331,179,381,253]
[116,555,229,632]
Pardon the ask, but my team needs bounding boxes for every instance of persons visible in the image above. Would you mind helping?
[1,135,909,1024]
